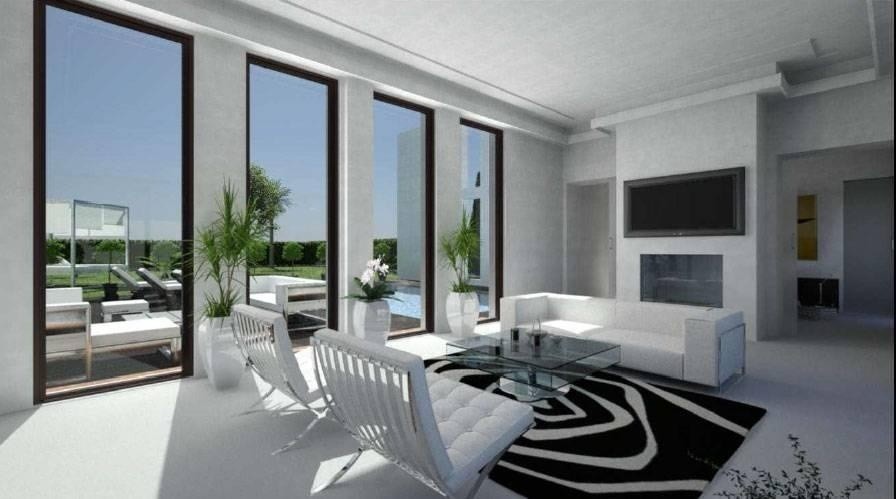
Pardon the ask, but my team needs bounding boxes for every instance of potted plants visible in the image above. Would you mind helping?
[183,179,268,391]
[440,203,486,337]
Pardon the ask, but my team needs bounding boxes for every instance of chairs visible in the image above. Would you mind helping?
[47,263,183,387]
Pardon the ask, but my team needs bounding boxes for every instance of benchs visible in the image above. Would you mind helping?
[294,327,535,497]
[232,305,343,456]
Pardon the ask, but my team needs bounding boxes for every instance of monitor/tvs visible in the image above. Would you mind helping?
[640,253,723,308]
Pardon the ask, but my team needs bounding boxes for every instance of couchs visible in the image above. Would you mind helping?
[250,275,327,333]
[500,291,744,395]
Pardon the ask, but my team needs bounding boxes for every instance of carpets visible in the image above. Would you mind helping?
[421,344,768,499]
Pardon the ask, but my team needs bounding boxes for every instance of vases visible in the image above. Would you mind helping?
[354,301,392,347]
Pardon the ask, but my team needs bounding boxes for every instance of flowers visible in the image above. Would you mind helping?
[341,253,405,303]
